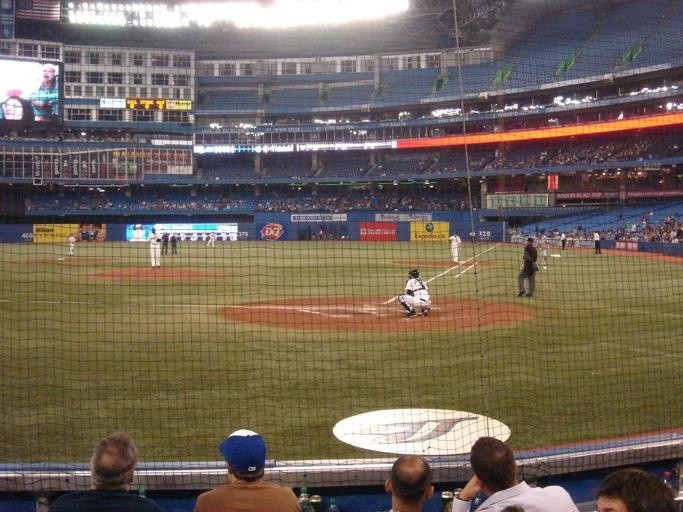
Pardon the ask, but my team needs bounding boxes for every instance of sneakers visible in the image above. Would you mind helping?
[407,309,428,316]
[517,290,532,296]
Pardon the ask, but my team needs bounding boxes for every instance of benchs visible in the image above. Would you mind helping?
[0,0,683,236]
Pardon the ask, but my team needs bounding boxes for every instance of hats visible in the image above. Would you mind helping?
[219,429,264,472]
[527,238,533,241]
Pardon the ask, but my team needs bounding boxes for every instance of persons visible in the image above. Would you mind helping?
[592,231,601,255]
[535,233,550,270]
[158,232,167,256]
[26,64,58,120]
[169,235,178,254]
[514,238,538,297]
[66,234,76,255]
[397,269,432,317]
[448,232,461,263]
[44,430,166,512]
[144,228,162,269]
[378,455,439,512]
[446,433,578,511]
[190,427,305,512]
[0,74,682,250]
[592,466,673,511]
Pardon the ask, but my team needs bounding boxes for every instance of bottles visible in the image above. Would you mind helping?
[470,492,483,512]
[298,486,310,512]
[138,487,146,499]
[327,498,339,512]
[660,468,679,498]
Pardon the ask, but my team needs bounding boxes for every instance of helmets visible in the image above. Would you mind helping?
[409,269,418,277]
